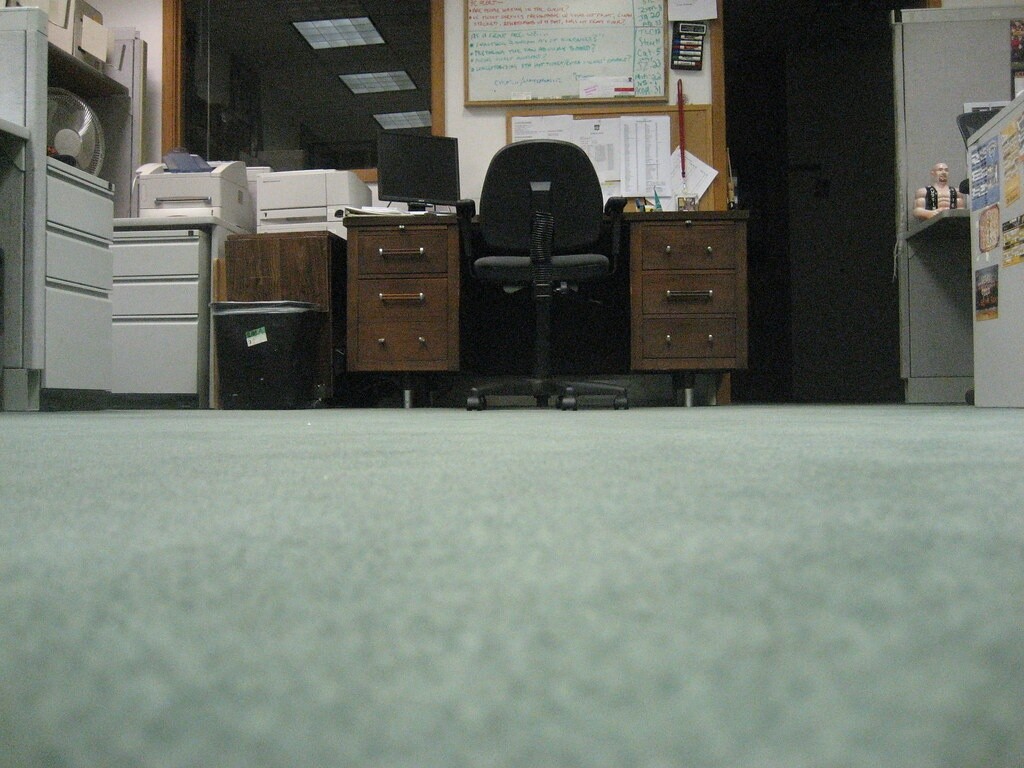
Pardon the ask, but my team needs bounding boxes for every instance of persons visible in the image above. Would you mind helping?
[912,163,964,220]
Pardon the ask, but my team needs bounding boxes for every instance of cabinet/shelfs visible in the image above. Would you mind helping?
[223,230,373,409]
[112,214,244,410]
[41,157,117,396]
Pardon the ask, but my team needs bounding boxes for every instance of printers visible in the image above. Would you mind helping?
[0,0,104,75]
[135,153,373,240]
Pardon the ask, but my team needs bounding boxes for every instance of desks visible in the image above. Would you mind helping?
[905,209,972,240]
[342,208,753,409]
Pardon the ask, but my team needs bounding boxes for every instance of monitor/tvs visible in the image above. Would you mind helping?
[375,128,461,212]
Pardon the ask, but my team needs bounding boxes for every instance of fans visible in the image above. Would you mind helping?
[46,87,106,179]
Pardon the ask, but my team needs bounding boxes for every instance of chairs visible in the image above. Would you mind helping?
[465,138,632,412]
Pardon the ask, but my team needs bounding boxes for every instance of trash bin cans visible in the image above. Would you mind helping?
[209,300,333,411]
